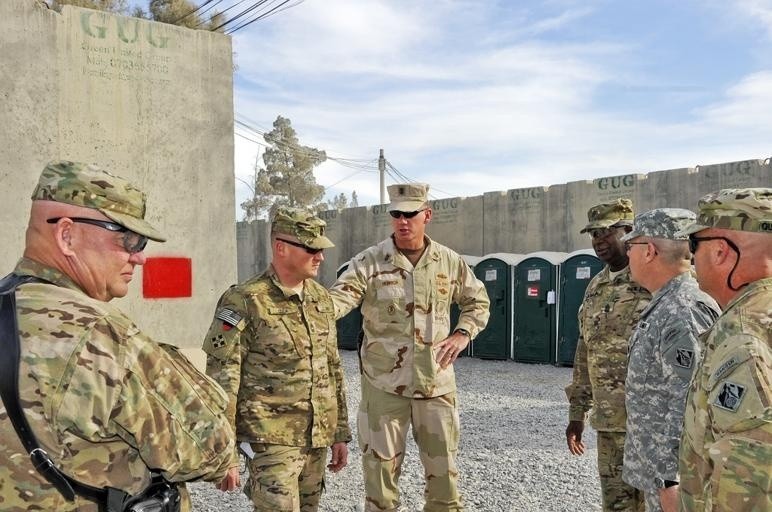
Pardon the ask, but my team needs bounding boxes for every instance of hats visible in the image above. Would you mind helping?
[29,157,168,245]
[386,183,430,213]
[579,197,634,234]
[673,188,772,238]
[270,205,335,251]
[619,208,697,241]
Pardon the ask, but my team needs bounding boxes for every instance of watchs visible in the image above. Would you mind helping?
[456,329,470,336]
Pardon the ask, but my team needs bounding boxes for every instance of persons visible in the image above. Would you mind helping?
[327,184,490,512]
[673,187,772,511]
[566,199,652,512]
[202,206,353,512]
[0,159,234,512]
[618,208,721,511]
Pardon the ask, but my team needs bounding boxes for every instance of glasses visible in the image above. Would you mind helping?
[624,240,658,256]
[45,215,148,254]
[389,211,420,218]
[275,238,323,255]
[588,226,622,239]
[687,234,739,254]
